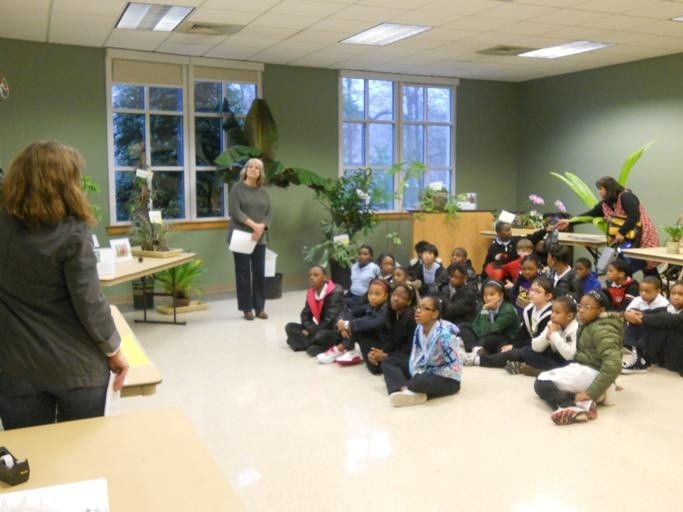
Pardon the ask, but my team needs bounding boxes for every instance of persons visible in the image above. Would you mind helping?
[380,296,466,407]
[1,141,128,433]
[284,264,342,357]
[224,156,272,321]
[312,277,389,367]
[558,178,661,278]
[358,284,416,376]
[532,287,627,426]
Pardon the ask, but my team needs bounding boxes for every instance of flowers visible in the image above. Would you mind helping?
[554,200,566,213]
[529,194,544,218]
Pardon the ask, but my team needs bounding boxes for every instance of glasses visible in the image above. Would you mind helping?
[576,304,600,312]
[415,305,435,312]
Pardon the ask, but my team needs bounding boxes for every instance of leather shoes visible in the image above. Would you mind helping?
[256,312,268,319]
[244,311,254,321]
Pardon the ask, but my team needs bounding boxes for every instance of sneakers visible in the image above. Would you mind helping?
[550,399,597,425]
[336,349,363,366]
[316,344,345,364]
[620,346,653,374]
[390,388,427,406]
[462,346,484,366]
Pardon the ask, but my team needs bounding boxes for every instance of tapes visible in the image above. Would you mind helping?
[0,453,14,469]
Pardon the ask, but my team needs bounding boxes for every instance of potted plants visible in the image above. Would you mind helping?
[307,167,385,289]
[123,170,172,310]
[417,185,447,213]
[153,248,206,306]
[219,94,331,300]
[660,223,682,254]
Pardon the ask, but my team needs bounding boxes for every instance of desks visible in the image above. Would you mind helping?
[406,209,499,279]
[622,246,682,267]
[481,228,608,277]
[95,248,198,329]
[0,405,239,510]
[103,304,163,398]
